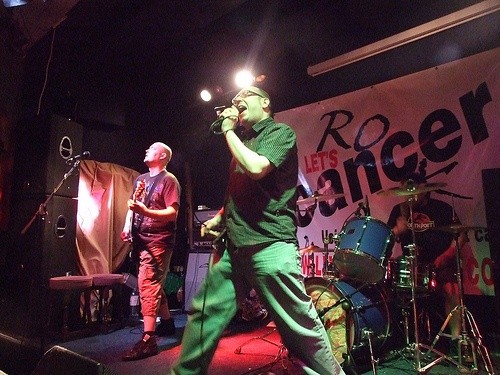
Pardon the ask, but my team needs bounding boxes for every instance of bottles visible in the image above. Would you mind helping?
[130,287,139,318]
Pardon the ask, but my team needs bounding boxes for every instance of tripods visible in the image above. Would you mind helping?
[379,194,496,375]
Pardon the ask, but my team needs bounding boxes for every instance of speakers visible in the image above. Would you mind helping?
[0,273,81,338]
[5,196,78,289]
[10,113,83,198]
[181,249,215,313]
[30,344,120,375]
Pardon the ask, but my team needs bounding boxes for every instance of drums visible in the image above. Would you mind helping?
[332,214,397,284]
[385,260,437,292]
[303,275,392,373]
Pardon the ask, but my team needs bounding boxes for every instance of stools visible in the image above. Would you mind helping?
[92,273,124,334]
[49,275,93,340]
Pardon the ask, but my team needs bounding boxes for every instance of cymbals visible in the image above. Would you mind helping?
[296,193,346,206]
[430,224,489,234]
[375,181,448,199]
[297,246,335,252]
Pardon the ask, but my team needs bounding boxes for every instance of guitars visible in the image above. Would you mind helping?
[127,177,146,273]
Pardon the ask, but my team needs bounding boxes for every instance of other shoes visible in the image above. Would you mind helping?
[157,317,176,336]
[441,339,458,367]
[122,332,157,360]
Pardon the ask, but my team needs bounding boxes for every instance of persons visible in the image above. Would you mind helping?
[167,87,346,374]
[120,141,182,360]
[388,173,467,359]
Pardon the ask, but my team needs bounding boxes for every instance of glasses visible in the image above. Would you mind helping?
[231,88,265,104]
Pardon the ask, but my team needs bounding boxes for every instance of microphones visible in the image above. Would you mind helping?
[66,151,91,163]
[213,106,242,128]
